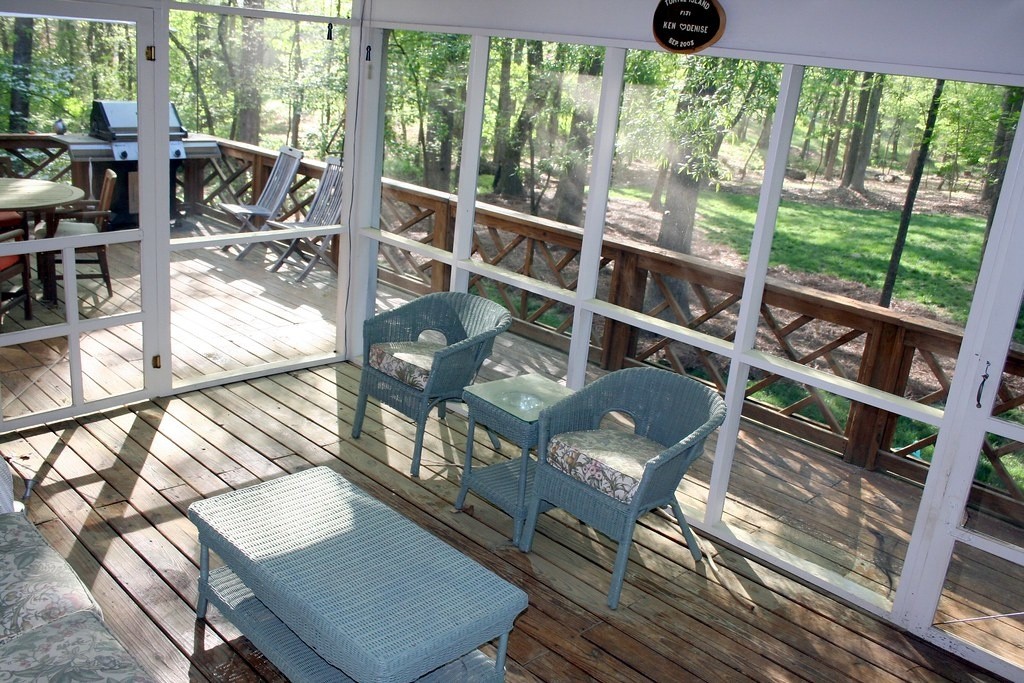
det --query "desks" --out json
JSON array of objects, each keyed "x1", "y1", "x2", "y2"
[
  {"x1": 455, "y1": 372, "x2": 586, "y2": 546},
  {"x1": 0, "y1": 177, "x2": 85, "y2": 300}
]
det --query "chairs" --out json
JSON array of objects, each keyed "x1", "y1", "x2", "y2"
[
  {"x1": 352, "y1": 292, "x2": 511, "y2": 476},
  {"x1": 0, "y1": 229, "x2": 33, "y2": 320},
  {"x1": 266, "y1": 156, "x2": 345, "y2": 280},
  {"x1": 218, "y1": 144, "x2": 304, "y2": 261},
  {"x1": 0, "y1": 157, "x2": 32, "y2": 277},
  {"x1": 39, "y1": 167, "x2": 117, "y2": 305},
  {"x1": 516, "y1": 367, "x2": 727, "y2": 610}
]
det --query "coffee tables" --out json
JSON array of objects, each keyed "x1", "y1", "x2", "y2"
[{"x1": 189, "y1": 465, "x2": 529, "y2": 683}]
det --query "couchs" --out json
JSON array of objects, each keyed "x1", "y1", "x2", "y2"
[{"x1": 0, "y1": 455, "x2": 159, "y2": 683}]
